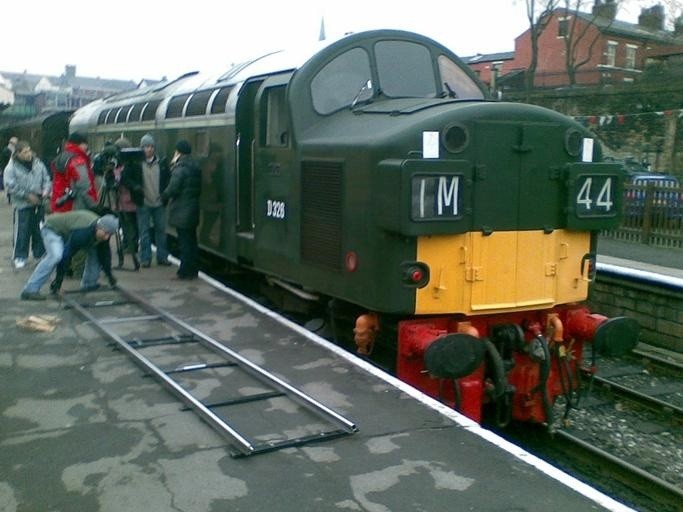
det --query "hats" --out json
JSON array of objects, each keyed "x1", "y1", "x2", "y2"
[
  {"x1": 114, "y1": 135, "x2": 155, "y2": 149},
  {"x1": 97, "y1": 214, "x2": 120, "y2": 233},
  {"x1": 69, "y1": 131, "x2": 87, "y2": 144},
  {"x1": 175, "y1": 139, "x2": 191, "y2": 154}
]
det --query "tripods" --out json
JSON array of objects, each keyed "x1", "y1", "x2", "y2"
[{"x1": 99, "y1": 168, "x2": 141, "y2": 272}]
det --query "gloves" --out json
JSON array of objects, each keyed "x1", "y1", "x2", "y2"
[
  {"x1": 109, "y1": 277, "x2": 117, "y2": 287},
  {"x1": 50, "y1": 276, "x2": 62, "y2": 293}
]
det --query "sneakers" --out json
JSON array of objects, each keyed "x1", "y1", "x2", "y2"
[
  {"x1": 14, "y1": 257, "x2": 26, "y2": 268},
  {"x1": 141, "y1": 258, "x2": 171, "y2": 267}
]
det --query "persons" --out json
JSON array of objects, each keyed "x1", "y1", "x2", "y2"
[
  {"x1": 21, "y1": 209, "x2": 119, "y2": 300},
  {"x1": 0, "y1": 131, "x2": 201, "y2": 279}
]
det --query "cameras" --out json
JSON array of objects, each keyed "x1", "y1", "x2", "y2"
[{"x1": 55, "y1": 187, "x2": 76, "y2": 207}]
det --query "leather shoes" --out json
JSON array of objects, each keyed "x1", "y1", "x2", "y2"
[
  {"x1": 21, "y1": 292, "x2": 47, "y2": 301},
  {"x1": 81, "y1": 284, "x2": 100, "y2": 292}
]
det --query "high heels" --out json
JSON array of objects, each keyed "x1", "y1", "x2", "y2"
[
  {"x1": 177, "y1": 270, "x2": 198, "y2": 280},
  {"x1": 115, "y1": 263, "x2": 140, "y2": 272}
]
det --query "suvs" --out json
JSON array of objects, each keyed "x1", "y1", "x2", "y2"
[{"x1": 618, "y1": 176, "x2": 682, "y2": 226}]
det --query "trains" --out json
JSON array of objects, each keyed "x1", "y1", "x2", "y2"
[{"x1": 0, "y1": 25, "x2": 645, "y2": 436}]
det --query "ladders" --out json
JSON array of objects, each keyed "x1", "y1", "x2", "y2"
[{"x1": 57, "y1": 282, "x2": 360, "y2": 458}]
